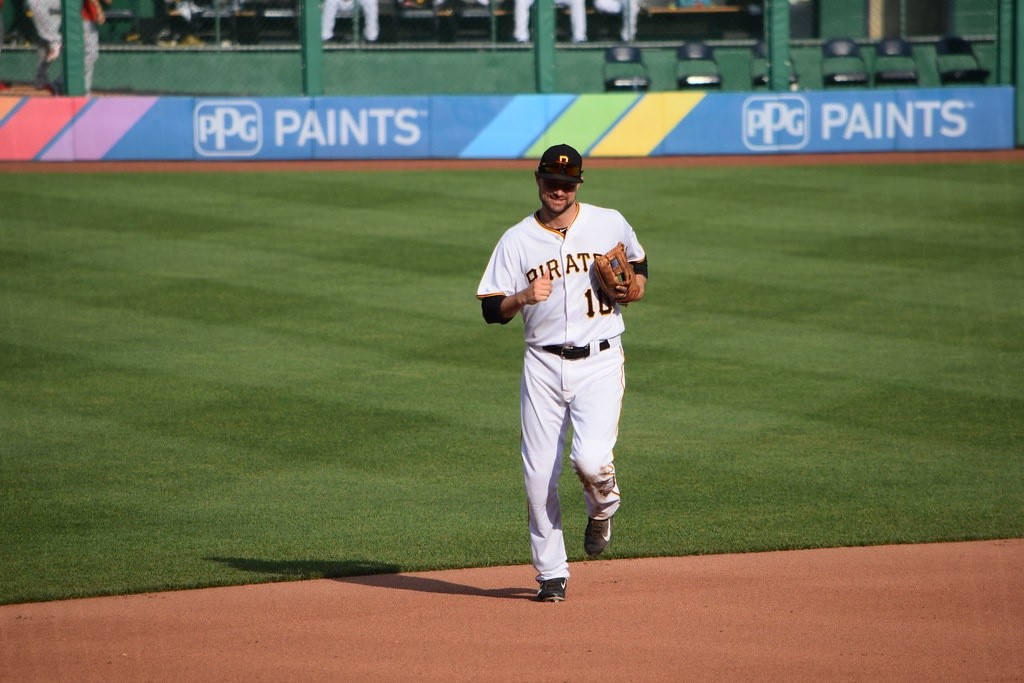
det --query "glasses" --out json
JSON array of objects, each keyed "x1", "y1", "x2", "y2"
[{"x1": 540, "y1": 162, "x2": 582, "y2": 177}]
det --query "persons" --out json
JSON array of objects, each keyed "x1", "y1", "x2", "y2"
[
  {"x1": 476, "y1": 144, "x2": 648, "y2": 603},
  {"x1": 0, "y1": 0, "x2": 111, "y2": 97},
  {"x1": 121, "y1": 0, "x2": 380, "y2": 47},
  {"x1": 514, "y1": 0, "x2": 648, "y2": 43}
]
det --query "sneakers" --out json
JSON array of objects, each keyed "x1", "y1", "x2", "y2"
[
  {"x1": 538, "y1": 577, "x2": 566, "y2": 601},
  {"x1": 584, "y1": 517, "x2": 612, "y2": 555}
]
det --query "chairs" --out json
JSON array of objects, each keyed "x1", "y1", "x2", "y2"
[
  {"x1": 874, "y1": 37, "x2": 919, "y2": 88},
  {"x1": 673, "y1": 41, "x2": 723, "y2": 91},
  {"x1": 99, "y1": 1, "x2": 764, "y2": 41},
  {"x1": 745, "y1": 42, "x2": 801, "y2": 92},
  {"x1": 603, "y1": 45, "x2": 649, "y2": 92},
  {"x1": 821, "y1": 39, "x2": 870, "y2": 88},
  {"x1": 933, "y1": 36, "x2": 987, "y2": 84}
]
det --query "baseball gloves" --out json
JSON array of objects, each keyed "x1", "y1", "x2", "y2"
[{"x1": 593, "y1": 243, "x2": 640, "y2": 305}]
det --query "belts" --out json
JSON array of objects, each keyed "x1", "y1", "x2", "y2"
[{"x1": 543, "y1": 340, "x2": 610, "y2": 360}]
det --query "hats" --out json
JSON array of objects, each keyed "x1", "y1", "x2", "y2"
[{"x1": 538, "y1": 143, "x2": 584, "y2": 183}]
